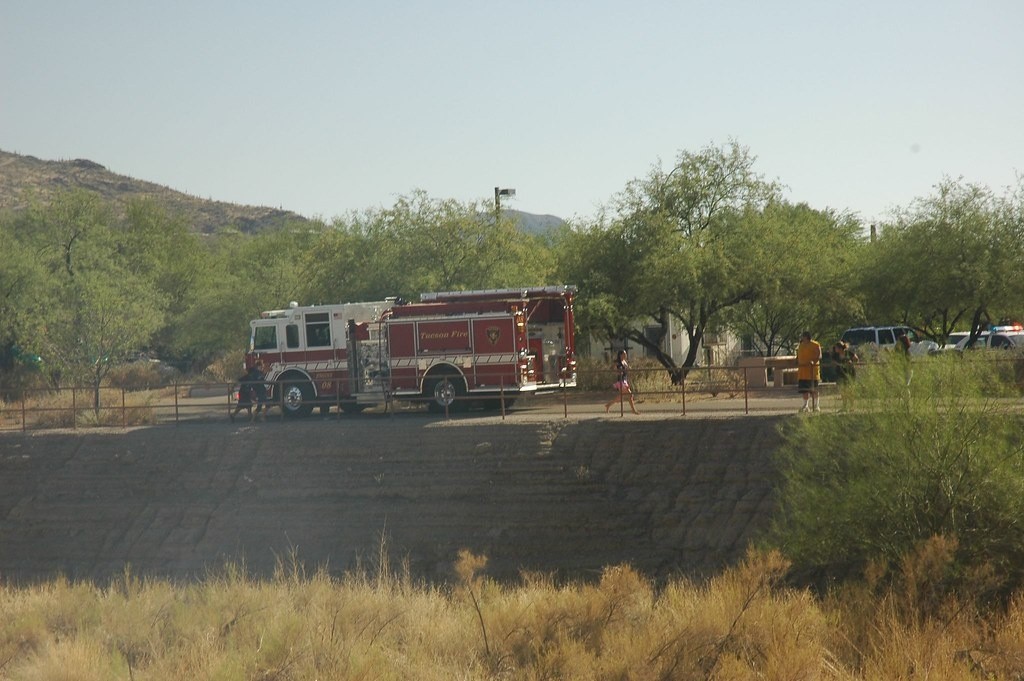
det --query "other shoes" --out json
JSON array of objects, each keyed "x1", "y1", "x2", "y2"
[
  {"x1": 814, "y1": 406, "x2": 820, "y2": 412},
  {"x1": 799, "y1": 407, "x2": 810, "y2": 412},
  {"x1": 230, "y1": 414, "x2": 235, "y2": 422}
]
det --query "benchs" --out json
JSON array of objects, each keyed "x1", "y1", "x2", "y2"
[{"x1": 773, "y1": 367, "x2": 798, "y2": 387}]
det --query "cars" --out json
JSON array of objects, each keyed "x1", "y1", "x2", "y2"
[{"x1": 945, "y1": 325, "x2": 1024, "y2": 351}]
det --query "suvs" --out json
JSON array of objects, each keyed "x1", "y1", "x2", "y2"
[{"x1": 840, "y1": 325, "x2": 939, "y2": 354}]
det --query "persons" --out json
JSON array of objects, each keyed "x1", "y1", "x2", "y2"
[
  {"x1": 832, "y1": 340, "x2": 859, "y2": 414},
  {"x1": 606, "y1": 350, "x2": 641, "y2": 416},
  {"x1": 253, "y1": 363, "x2": 270, "y2": 422},
  {"x1": 231, "y1": 367, "x2": 255, "y2": 424},
  {"x1": 796, "y1": 333, "x2": 821, "y2": 413},
  {"x1": 893, "y1": 334, "x2": 914, "y2": 402}
]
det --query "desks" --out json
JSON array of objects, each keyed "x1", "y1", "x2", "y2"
[{"x1": 738, "y1": 355, "x2": 798, "y2": 387}]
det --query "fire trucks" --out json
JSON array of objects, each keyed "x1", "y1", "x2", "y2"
[{"x1": 243, "y1": 284, "x2": 578, "y2": 417}]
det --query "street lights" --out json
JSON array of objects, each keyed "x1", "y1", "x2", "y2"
[{"x1": 493, "y1": 186, "x2": 517, "y2": 225}]
